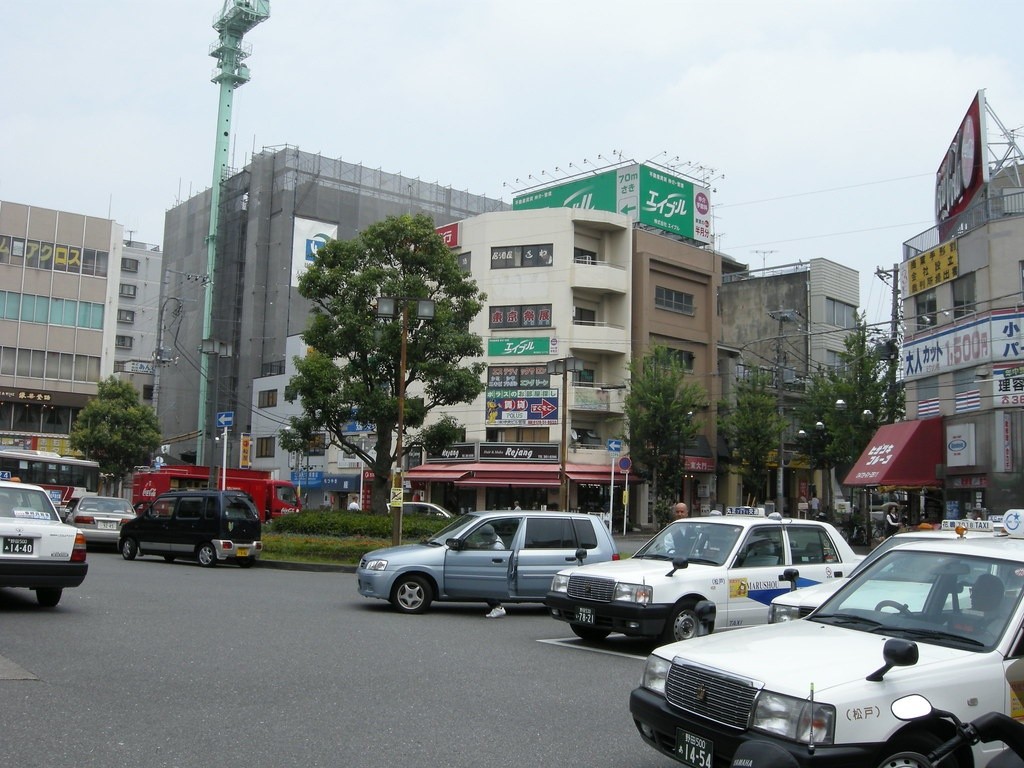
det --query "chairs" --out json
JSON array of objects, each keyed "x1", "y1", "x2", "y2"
[{"x1": 741, "y1": 541, "x2": 781, "y2": 567}]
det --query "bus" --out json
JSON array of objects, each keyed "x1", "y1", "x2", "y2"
[{"x1": 0, "y1": 446, "x2": 100, "y2": 520}]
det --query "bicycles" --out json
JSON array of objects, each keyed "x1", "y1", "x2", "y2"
[{"x1": 870, "y1": 517, "x2": 884, "y2": 539}]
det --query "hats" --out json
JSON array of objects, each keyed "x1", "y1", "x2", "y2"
[{"x1": 479, "y1": 523, "x2": 497, "y2": 540}]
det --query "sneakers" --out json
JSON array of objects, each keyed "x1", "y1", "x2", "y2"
[{"x1": 486, "y1": 606, "x2": 506, "y2": 617}]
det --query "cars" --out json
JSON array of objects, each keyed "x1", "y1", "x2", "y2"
[
  {"x1": 116, "y1": 488, "x2": 265, "y2": 568},
  {"x1": 834, "y1": 525, "x2": 879, "y2": 545},
  {"x1": 0, "y1": 481, "x2": 90, "y2": 608},
  {"x1": 385, "y1": 502, "x2": 454, "y2": 520},
  {"x1": 64, "y1": 496, "x2": 138, "y2": 545},
  {"x1": 767, "y1": 523, "x2": 1024, "y2": 625},
  {"x1": 628, "y1": 535, "x2": 1023, "y2": 768},
  {"x1": 541, "y1": 513, "x2": 894, "y2": 648},
  {"x1": 356, "y1": 508, "x2": 619, "y2": 615}
]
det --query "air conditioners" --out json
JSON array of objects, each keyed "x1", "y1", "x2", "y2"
[{"x1": 576, "y1": 255, "x2": 592, "y2": 264}]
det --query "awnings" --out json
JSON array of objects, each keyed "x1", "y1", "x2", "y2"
[
  {"x1": 401, "y1": 462, "x2": 647, "y2": 489},
  {"x1": 841, "y1": 416, "x2": 945, "y2": 490}
]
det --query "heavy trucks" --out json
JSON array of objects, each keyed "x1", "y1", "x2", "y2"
[{"x1": 131, "y1": 464, "x2": 301, "y2": 523}]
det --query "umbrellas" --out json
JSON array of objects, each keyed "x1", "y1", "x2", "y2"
[{"x1": 880, "y1": 501, "x2": 900, "y2": 508}]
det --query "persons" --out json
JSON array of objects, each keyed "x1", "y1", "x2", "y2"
[
  {"x1": 966, "y1": 509, "x2": 974, "y2": 519},
  {"x1": 475, "y1": 523, "x2": 516, "y2": 618},
  {"x1": 964, "y1": 573, "x2": 1013, "y2": 640},
  {"x1": 885, "y1": 504, "x2": 909, "y2": 540},
  {"x1": 513, "y1": 501, "x2": 522, "y2": 512},
  {"x1": 808, "y1": 492, "x2": 823, "y2": 520},
  {"x1": 663, "y1": 502, "x2": 710, "y2": 560},
  {"x1": 281, "y1": 493, "x2": 291, "y2": 505},
  {"x1": 348, "y1": 495, "x2": 360, "y2": 512},
  {"x1": 411, "y1": 491, "x2": 421, "y2": 503}
]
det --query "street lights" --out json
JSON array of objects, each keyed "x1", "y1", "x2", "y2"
[
  {"x1": 375, "y1": 296, "x2": 436, "y2": 546},
  {"x1": 546, "y1": 356, "x2": 584, "y2": 510}
]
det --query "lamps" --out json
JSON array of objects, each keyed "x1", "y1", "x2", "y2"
[
  {"x1": 598, "y1": 154, "x2": 613, "y2": 165},
  {"x1": 709, "y1": 173, "x2": 726, "y2": 183},
  {"x1": 938, "y1": 308, "x2": 978, "y2": 320},
  {"x1": 502, "y1": 181, "x2": 517, "y2": 193},
  {"x1": 583, "y1": 158, "x2": 598, "y2": 168},
  {"x1": 514, "y1": 178, "x2": 531, "y2": 188},
  {"x1": 698, "y1": 169, "x2": 716, "y2": 179},
  {"x1": 541, "y1": 170, "x2": 556, "y2": 181},
  {"x1": 554, "y1": 166, "x2": 570, "y2": 176},
  {"x1": 663, "y1": 155, "x2": 680, "y2": 165},
  {"x1": 675, "y1": 160, "x2": 692, "y2": 169},
  {"x1": 643, "y1": 150, "x2": 668, "y2": 165},
  {"x1": 688, "y1": 165, "x2": 704, "y2": 174},
  {"x1": 919, "y1": 314, "x2": 958, "y2": 327},
  {"x1": 710, "y1": 187, "x2": 717, "y2": 193},
  {"x1": 899, "y1": 316, "x2": 935, "y2": 335},
  {"x1": 613, "y1": 149, "x2": 628, "y2": 161},
  {"x1": 568, "y1": 162, "x2": 584, "y2": 173},
  {"x1": 528, "y1": 174, "x2": 544, "y2": 185},
  {"x1": 881, "y1": 323, "x2": 915, "y2": 340}
]
